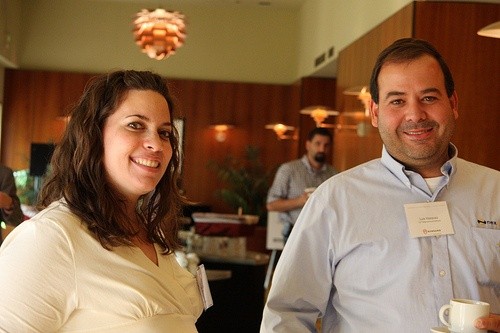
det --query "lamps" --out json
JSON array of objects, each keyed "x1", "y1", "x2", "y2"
[{"x1": 134, "y1": 8, "x2": 187, "y2": 61}]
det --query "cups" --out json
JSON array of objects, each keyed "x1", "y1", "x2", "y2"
[{"x1": 439, "y1": 297, "x2": 490, "y2": 333}]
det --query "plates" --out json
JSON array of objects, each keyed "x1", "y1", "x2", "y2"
[{"x1": 430, "y1": 327, "x2": 450, "y2": 333}]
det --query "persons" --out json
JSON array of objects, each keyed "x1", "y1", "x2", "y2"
[
  {"x1": 0, "y1": 70, "x2": 213, "y2": 333},
  {"x1": 263, "y1": 128, "x2": 341, "y2": 305},
  {"x1": 259, "y1": 38, "x2": 500, "y2": 333},
  {"x1": 0, "y1": 162, "x2": 25, "y2": 224}
]
